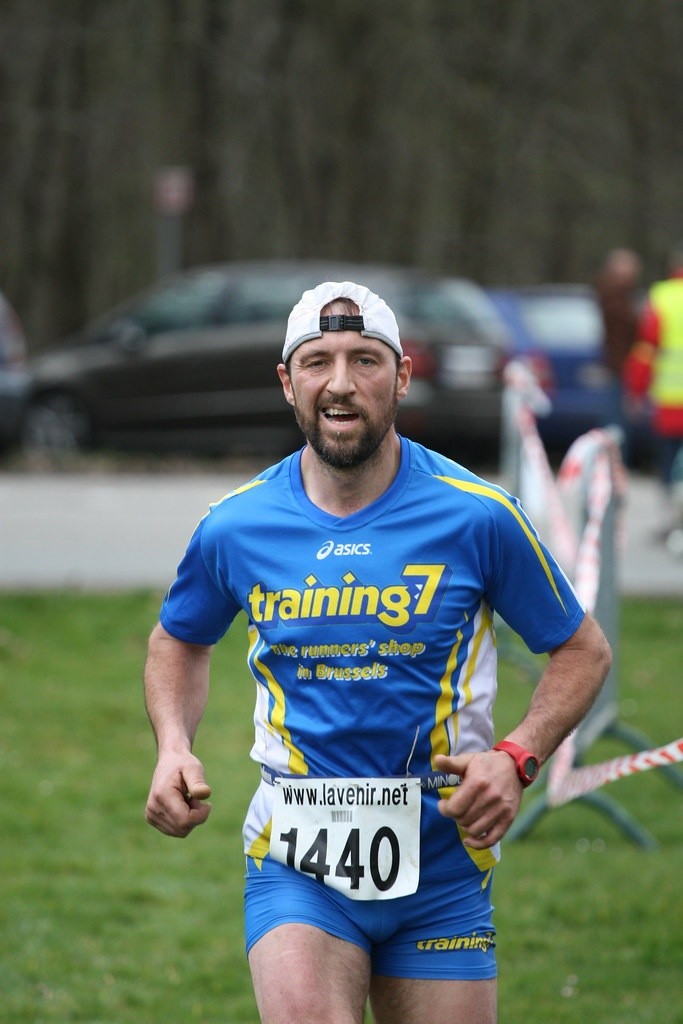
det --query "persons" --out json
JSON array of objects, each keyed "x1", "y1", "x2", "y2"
[
  {"x1": 141, "y1": 280, "x2": 615, "y2": 1024},
  {"x1": 598, "y1": 247, "x2": 683, "y2": 481}
]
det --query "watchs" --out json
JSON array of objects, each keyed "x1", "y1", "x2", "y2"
[{"x1": 494, "y1": 741, "x2": 540, "y2": 788}]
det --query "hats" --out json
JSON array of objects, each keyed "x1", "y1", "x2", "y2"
[{"x1": 281, "y1": 282, "x2": 404, "y2": 362}]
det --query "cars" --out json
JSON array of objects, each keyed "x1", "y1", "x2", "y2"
[{"x1": 492, "y1": 282, "x2": 635, "y2": 433}]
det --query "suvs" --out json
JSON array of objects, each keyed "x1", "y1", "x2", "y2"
[{"x1": 0, "y1": 258, "x2": 516, "y2": 465}]
